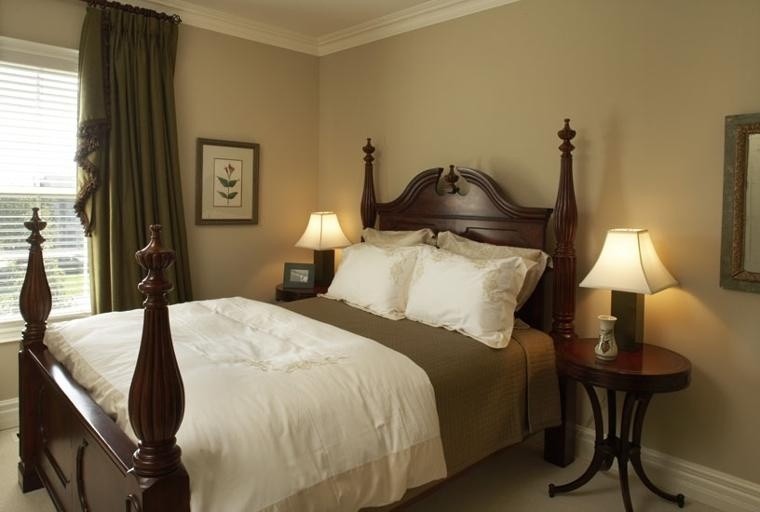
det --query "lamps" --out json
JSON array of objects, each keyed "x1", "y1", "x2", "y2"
[
  {"x1": 294, "y1": 210, "x2": 353, "y2": 288},
  {"x1": 578, "y1": 228, "x2": 680, "y2": 351}
]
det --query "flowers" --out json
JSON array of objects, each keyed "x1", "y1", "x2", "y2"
[{"x1": 216, "y1": 164, "x2": 239, "y2": 206}]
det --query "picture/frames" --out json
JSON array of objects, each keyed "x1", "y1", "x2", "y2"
[
  {"x1": 719, "y1": 112, "x2": 759, "y2": 294},
  {"x1": 195, "y1": 137, "x2": 260, "y2": 225},
  {"x1": 283, "y1": 261, "x2": 316, "y2": 288}
]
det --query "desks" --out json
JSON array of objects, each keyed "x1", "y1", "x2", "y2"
[
  {"x1": 276, "y1": 282, "x2": 331, "y2": 302},
  {"x1": 548, "y1": 338, "x2": 691, "y2": 512}
]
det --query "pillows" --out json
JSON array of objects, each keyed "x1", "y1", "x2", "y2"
[
  {"x1": 436, "y1": 231, "x2": 553, "y2": 330},
  {"x1": 406, "y1": 243, "x2": 538, "y2": 350},
  {"x1": 358, "y1": 227, "x2": 437, "y2": 248},
  {"x1": 316, "y1": 242, "x2": 425, "y2": 322}
]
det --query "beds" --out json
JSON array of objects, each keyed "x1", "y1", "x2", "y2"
[{"x1": 17, "y1": 118, "x2": 578, "y2": 512}]
